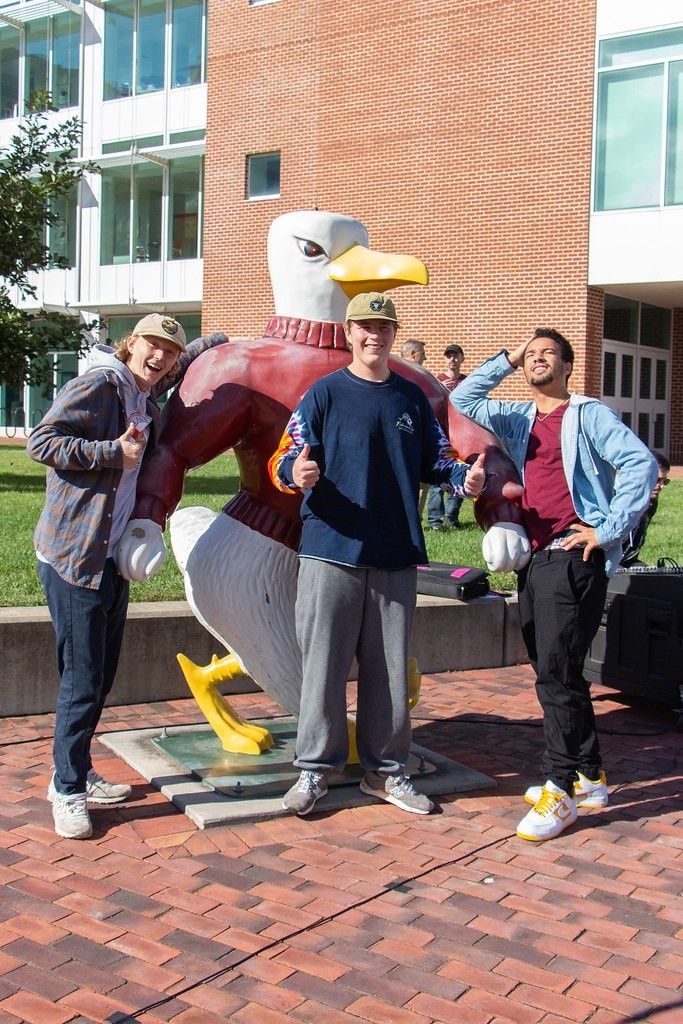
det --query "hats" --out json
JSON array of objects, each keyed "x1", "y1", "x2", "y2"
[
  {"x1": 132, "y1": 313, "x2": 187, "y2": 353},
  {"x1": 443, "y1": 344, "x2": 464, "y2": 356},
  {"x1": 346, "y1": 292, "x2": 400, "y2": 322}
]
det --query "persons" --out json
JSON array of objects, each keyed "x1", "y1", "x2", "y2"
[
  {"x1": 616, "y1": 448, "x2": 670, "y2": 570},
  {"x1": 401, "y1": 339, "x2": 431, "y2": 522},
  {"x1": 424, "y1": 344, "x2": 468, "y2": 534},
  {"x1": 449, "y1": 328, "x2": 659, "y2": 841},
  {"x1": 28, "y1": 311, "x2": 259, "y2": 837},
  {"x1": 268, "y1": 291, "x2": 500, "y2": 816}
]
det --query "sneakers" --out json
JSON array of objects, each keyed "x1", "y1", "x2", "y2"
[
  {"x1": 360, "y1": 770, "x2": 435, "y2": 815},
  {"x1": 524, "y1": 769, "x2": 608, "y2": 808},
  {"x1": 430, "y1": 522, "x2": 443, "y2": 532},
  {"x1": 283, "y1": 769, "x2": 329, "y2": 816},
  {"x1": 46, "y1": 766, "x2": 132, "y2": 804},
  {"x1": 445, "y1": 516, "x2": 461, "y2": 530},
  {"x1": 517, "y1": 779, "x2": 577, "y2": 841},
  {"x1": 51, "y1": 792, "x2": 92, "y2": 839}
]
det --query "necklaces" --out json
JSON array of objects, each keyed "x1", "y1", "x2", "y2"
[{"x1": 536, "y1": 395, "x2": 569, "y2": 422}]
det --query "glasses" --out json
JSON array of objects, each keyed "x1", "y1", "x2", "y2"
[{"x1": 657, "y1": 477, "x2": 670, "y2": 485}]
